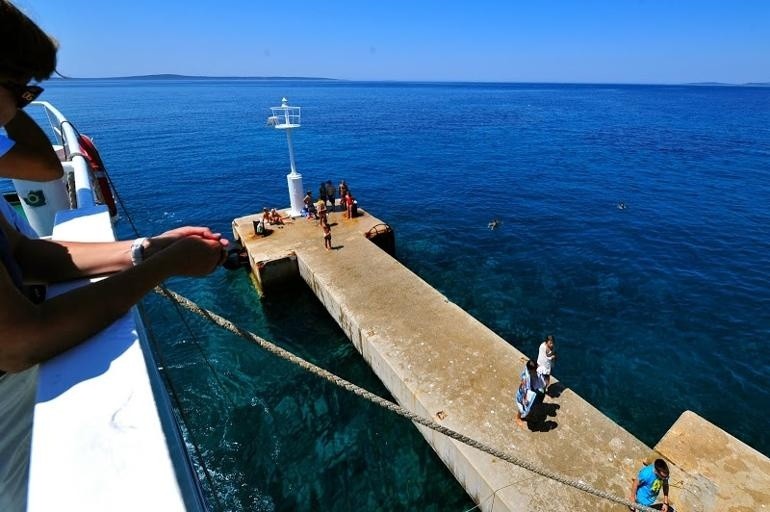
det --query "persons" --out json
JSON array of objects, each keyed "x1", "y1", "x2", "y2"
[
  {"x1": 0, "y1": 0, "x2": 230, "y2": 512},
  {"x1": 488, "y1": 218, "x2": 499, "y2": 230},
  {"x1": 0, "y1": 107, "x2": 64, "y2": 239},
  {"x1": 619, "y1": 203, "x2": 625, "y2": 210},
  {"x1": 302, "y1": 178, "x2": 353, "y2": 228},
  {"x1": 629, "y1": 459, "x2": 670, "y2": 512},
  {"x1": 261, "y1": 206, "x2": 294, "y2": 225},
  {"x1": 535, "y1": 334, "x2": 557, "y2": 386},
  {"x1": 514, "y1": 360, "x2": 546, "y2": 429},
  {"x1": 322, "y1": 218, "x2": 332, "y2": 250}
]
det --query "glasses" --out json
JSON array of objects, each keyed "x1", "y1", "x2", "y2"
[
  {"x1": 658, "y1": 472, "x2": 669, "y2": 480},
  {"x1": 16, "y1": 86, "x2": 44, "y2": 109}
]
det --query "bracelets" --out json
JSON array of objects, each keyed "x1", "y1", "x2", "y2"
[
  {"x1": 664, "y1": 502, "x2": 669, "y2": 507},
  {"x1": 130, "y1": 234, "x2": 145, "y2": 268}
]
